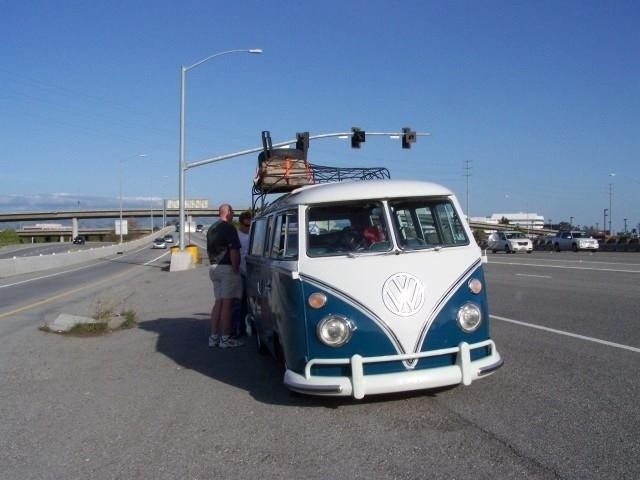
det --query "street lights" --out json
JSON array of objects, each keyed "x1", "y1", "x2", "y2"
[
  {"x1": 118, "y1": 154, "x2": 144, "y2": 242},
  {"x1": 149, "y1": 175, "x2": 168, "y2": 233},
  {"x1": 178, "y1": 47, "x2": 262, "y2": 250}
]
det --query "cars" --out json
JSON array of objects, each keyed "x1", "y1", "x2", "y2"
[
  {"x1": 164, "y1": 235, "x2": 172, "y2": 243},
  {"x1": 153, "y1": 238, "x2": 166, "y2": 249},
  {"x1": 196, "y1": 224, "x2": 203, "y2": 231},
  {"x1": 488, "y1": 230, "x2": 533, "y2": 254},
  {"x1": 551, "y1": 230, "x2": 599, "y2": 251},
  {"x1": 73, "y1": 236, "x2": 86, "y2": 245}
]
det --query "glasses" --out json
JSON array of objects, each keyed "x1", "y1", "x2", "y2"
[{"x1": 243, "y1": 223, "x2": 250, "y2": 227}]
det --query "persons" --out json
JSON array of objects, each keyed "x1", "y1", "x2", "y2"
[
  {"x1": 207, "y1": 204, "x2": 245, "y2": 348},
  {"x1": 229, "y1": 212, "x2": 252, "y2": 339}
]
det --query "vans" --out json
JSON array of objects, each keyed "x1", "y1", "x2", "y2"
[{"x1": 242, "y1": 178, "x2": 505, "y2": 398}]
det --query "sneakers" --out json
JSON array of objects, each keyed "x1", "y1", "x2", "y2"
[
  {"x1": 219, "y1": 335, "x2": 245, "y2": 348},
  {"x1": 209, "y1": 335, "x2": 220, "y2": 348},
  {"x1": 239, "y1": 331, "x2": 248, "y2": 336},
  {"x1": 231, "y1": 330, "x2": 237, "y2": 338}
]
